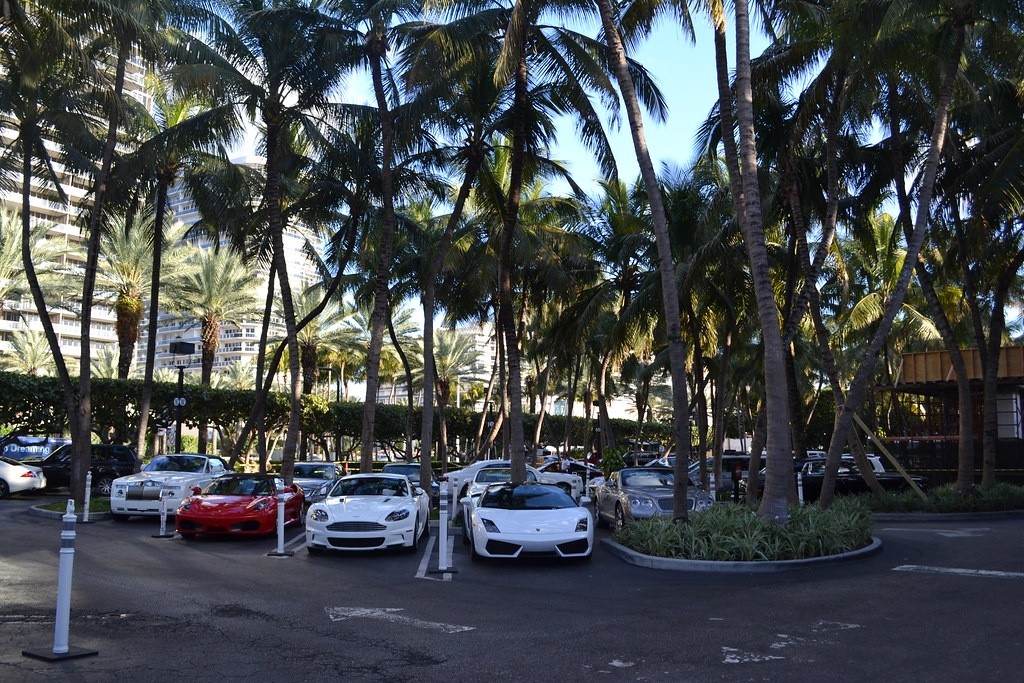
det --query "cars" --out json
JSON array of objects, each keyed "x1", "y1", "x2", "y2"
[{"x1": 0, "y1": 435, "x2": 929, "y2": 565}]
